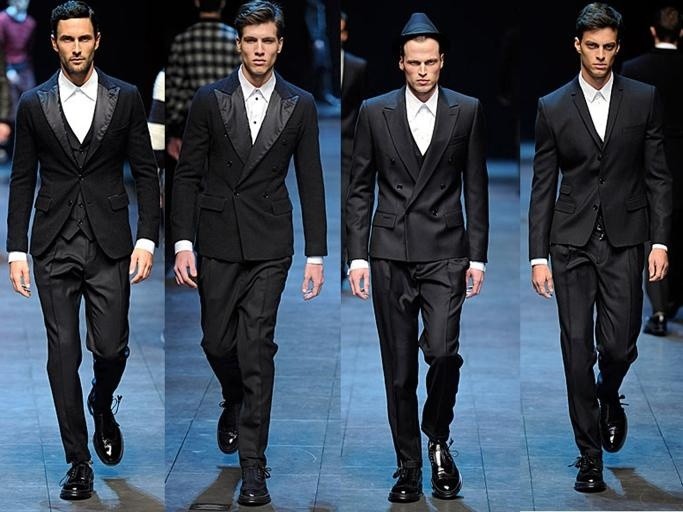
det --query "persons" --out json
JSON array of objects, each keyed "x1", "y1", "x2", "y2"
[
  {"x1": 526, "y1": 1, "x2": 674, "y2": 489},
  {"x1": 340, "y1": 15, "x2": 372, "y2": 284},
  {"x1": 344, "y1": 34, "x2": 492, "y2": 503},
  {"x1": 164, "y1": 1, "x2": 243, "y2": 233},
  {"x1": 619, "y1": 3, "x2": 682, "y2": 338},
  {"x1": 3, "y1": 0, "x2": 161, "y2": 502},
  {"x1": 169, "y1": 0, "x2": 329, "y2": 508},
  {"x1": 0, "y1": 0, "x2": 37, "y2": 157}
]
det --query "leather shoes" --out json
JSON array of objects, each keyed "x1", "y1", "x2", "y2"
[
  {"x1": 87, "y1": 393, "x2": 124, "y2": 465},
  {"x1": 387, "y1": 466, "x2": 424, "y2": 504},
  {"x1": 216, "y1": 399, "x2": 239, "y2": 454},
  {"x1": 599, "y1": 390, "x2": 628, "y2": 452},
  {"x1": 236, "y1": 466, "x2": 270, "y2": 507},
  {"x1": 427, "y1": 438, "x2": 464, "y2": 499},
  {"x1": 574, "y1": 454, "x2": 608, "y2": 495},
  {"x1": 59, "y1": 462, "x2": 95, "y2": 501},
  {"x1": 643, "y1": 310, "x2": 669, "y2": 335}
]
